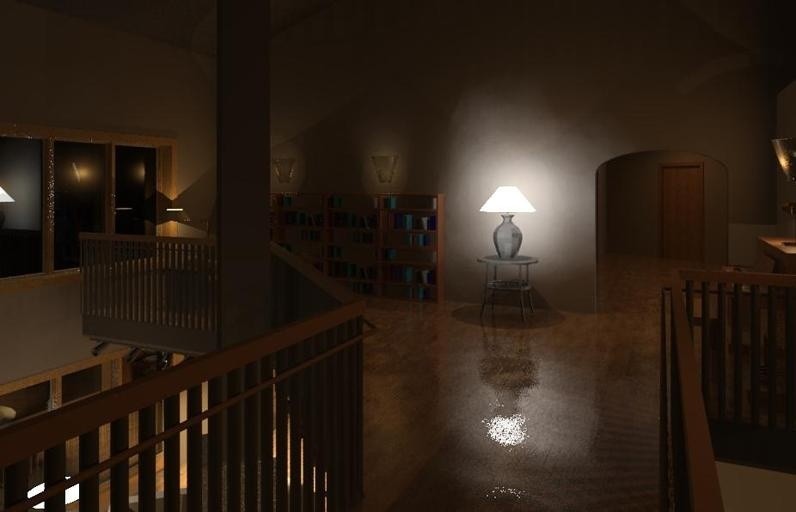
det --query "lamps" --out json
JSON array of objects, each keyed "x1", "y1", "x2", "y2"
[
  {"x1": 0, "y1": 186, "x2": 15, "y2": 231},
  {"x1": 272, "y1": 158, "x2": 296, "y2": 183},
  {"x1": 157, "y1": 207, "x2": 192, "y2": 248},
  {"x1": 769, "y1": 136, "x2": 796, "y2": 182},
  {"x1": 371, "y1": 153, "x2": 401, "y2": 183},
  {"x1": 479, "y1": 185, "x2": 537, "y2": 259}
]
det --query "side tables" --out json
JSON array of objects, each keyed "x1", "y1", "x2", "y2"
[{"x1": 475, "y1": 255, "x2": 539, "y2": 323}]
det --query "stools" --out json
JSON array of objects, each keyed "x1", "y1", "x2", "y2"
[{"x1": 720, "y1": 265, "x2": 757, "y2": 330}]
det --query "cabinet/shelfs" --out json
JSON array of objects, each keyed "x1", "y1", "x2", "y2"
[{"x1": 270, "y1": 191, "x2": 446, "y2": 307}]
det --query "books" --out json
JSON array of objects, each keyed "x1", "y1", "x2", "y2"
[
  {"x1": 278, "y1": 192, "x2": 323, "y2": 257},
  {"x1": 382, "y1": 195, "x2": 437, "y2": 302},
  {"x1": 328, "y1": 193, "x2": 379, "y2": 296}
]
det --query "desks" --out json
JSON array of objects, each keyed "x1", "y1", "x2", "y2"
[{"x1": 757, "y1": 234, "x2": 796, "y2": 298}]
